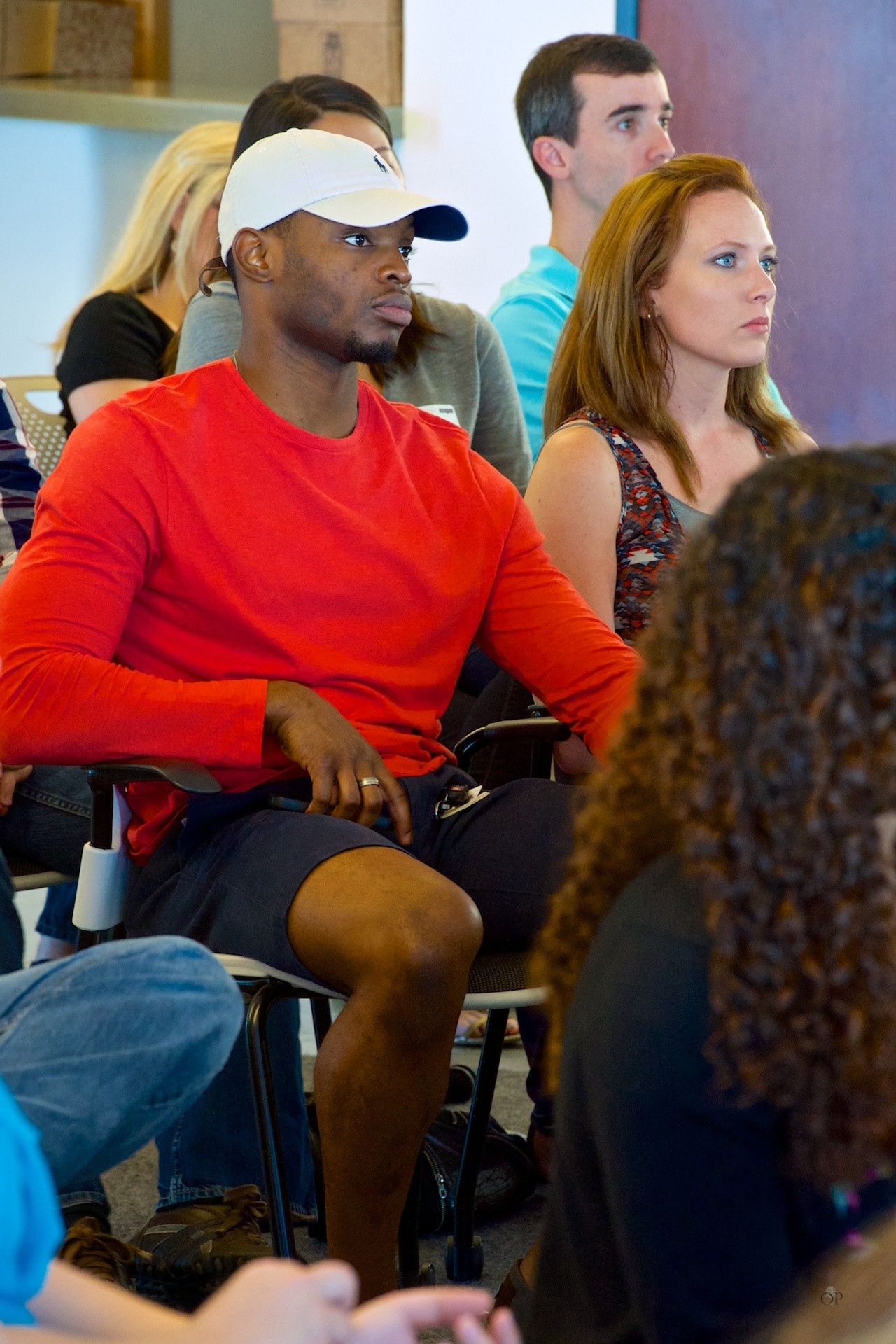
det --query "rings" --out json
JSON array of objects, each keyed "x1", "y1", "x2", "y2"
[{"x1": 358, "y1": 776, "x2": 381, "y2": 788}]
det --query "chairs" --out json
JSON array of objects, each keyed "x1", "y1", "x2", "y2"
[{"x1": 0, "y1": 376, "x2": 574, "y2": 1278}]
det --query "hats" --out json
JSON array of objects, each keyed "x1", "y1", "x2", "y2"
[{"x1": 216, "y1": 127, "x2": 469, "y2": 267}]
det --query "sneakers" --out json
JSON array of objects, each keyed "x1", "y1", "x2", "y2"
[
  {"x1": 127, "y1": 1176, "x2": 318, "y2": 1301},
  {"x1": 55, "y1": 1216, "x2": 162, "y2": 1304}
]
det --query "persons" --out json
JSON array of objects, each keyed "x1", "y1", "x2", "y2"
[{"x1": 0, "y1": 33, "x2": 895, "y2": 1343}]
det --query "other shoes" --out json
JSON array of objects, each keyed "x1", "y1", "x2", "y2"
[{"x1": 496, "y1": 1259, "x2": 568, "y2": 1344}]
